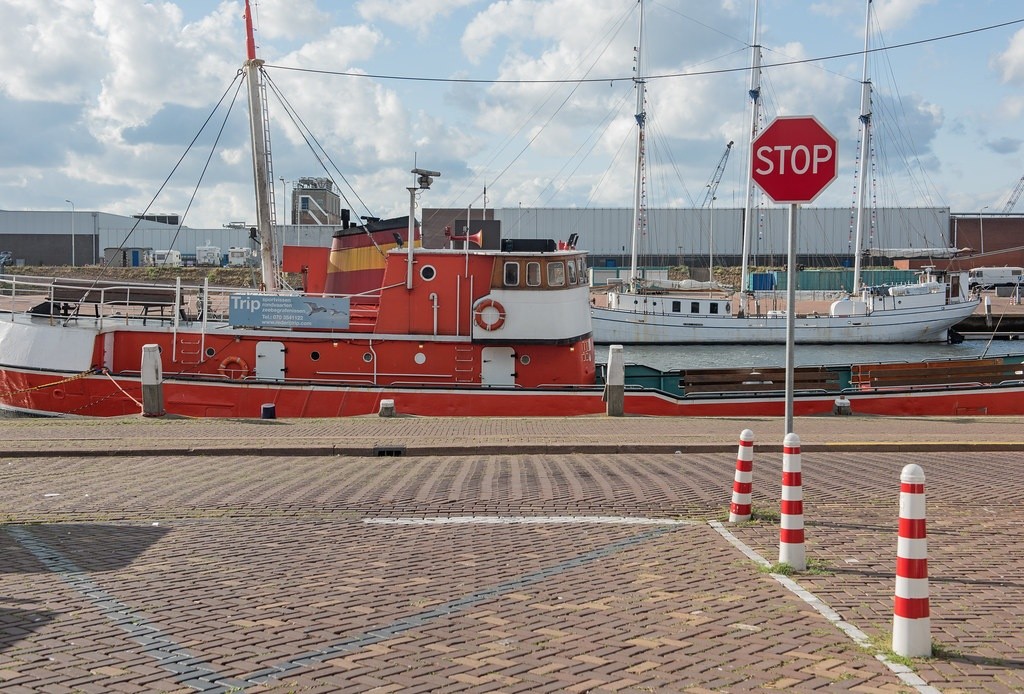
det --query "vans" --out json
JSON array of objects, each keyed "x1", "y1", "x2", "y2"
[{"x1": 969, "y1": 266, "x2": 1024, "y2": 291}]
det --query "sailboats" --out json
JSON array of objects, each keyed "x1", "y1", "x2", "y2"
[{"x1": 589, "y1": 0, "x2": 985, "y2": 347}]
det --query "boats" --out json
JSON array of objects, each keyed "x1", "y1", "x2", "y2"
[{"x1": 0, "y1": 0, "x2": 1024, "y2": 421}]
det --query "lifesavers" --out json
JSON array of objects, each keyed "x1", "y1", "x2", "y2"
[
  {"x1": 474, "y1": 299, "x2": 506, "y2": 332},
  {"x1": 217, "y1": 355, "x2": 249, "y2": 384}
]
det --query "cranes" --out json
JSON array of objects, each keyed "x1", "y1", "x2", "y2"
[
  {"x1": 1001, "y1": 174, "x2": 1024, "y2": 214},
  {"x1": 696, "y1": 140, "x2": 734, "y2": 208}
]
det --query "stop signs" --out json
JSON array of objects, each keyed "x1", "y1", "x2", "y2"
[{"x1": 750, "y1": 115, "x2": 839, "y2": 204}]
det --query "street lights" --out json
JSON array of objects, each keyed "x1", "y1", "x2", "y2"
[
  {"x1": 65, "y1": 199, "x2": 75, "y2": 267},
  {"x1": 980, "y1": 206, "x2": 989, "y2": 255}
]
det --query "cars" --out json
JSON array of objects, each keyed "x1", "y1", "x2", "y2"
[{"x1": 0, "y1": 250, "x2": 14, "y2": 266}]
